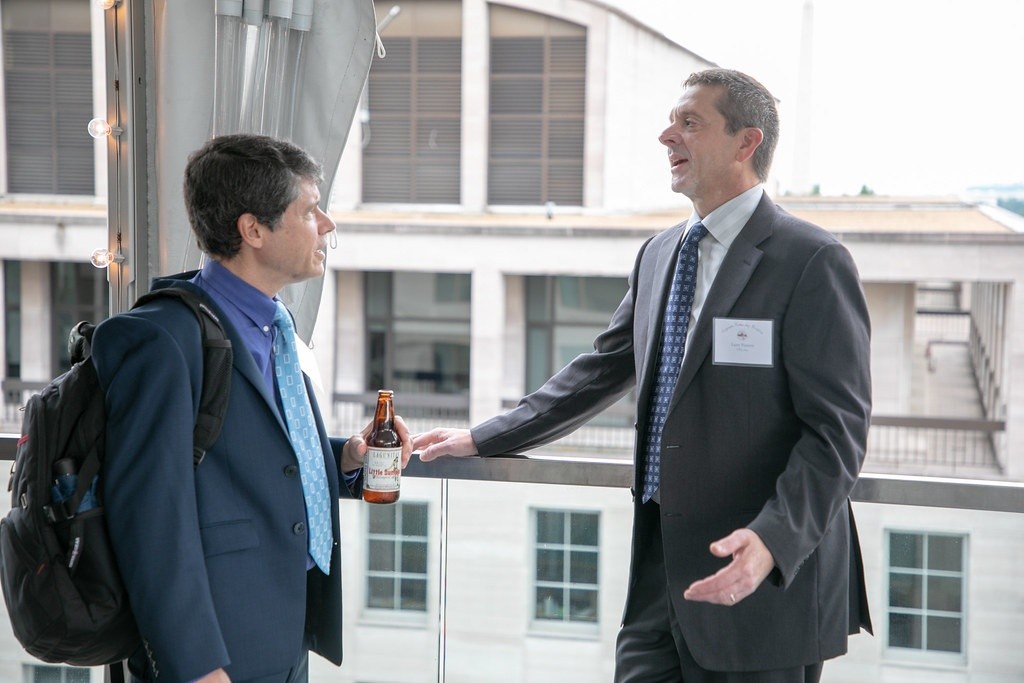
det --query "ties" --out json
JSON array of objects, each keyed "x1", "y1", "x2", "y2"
[
  {"x1": 268, "y1": 301, "x2": 335, "y2": 576},
  {"x1": 640, "y1": 222, "x2": 709, "y2": 504}
]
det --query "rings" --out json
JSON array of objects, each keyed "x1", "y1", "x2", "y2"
[{"x1": 730, "y1": 594, "x2": 735, "y2": 602}]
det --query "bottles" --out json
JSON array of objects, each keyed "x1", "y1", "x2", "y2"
[{"x1": 362, "y1": 390, "x2": 402, "y2": 503}]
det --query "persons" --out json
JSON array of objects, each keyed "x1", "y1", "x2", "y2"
[
  {"x1": 412, "y1": 69, "x2": 874, "y2": 683},
  {"x1": 91, "y1": 135, "x2": 414, "y2": 683}
]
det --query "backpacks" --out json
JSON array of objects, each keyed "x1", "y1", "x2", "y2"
[{"x1": 0, "y1": 286, "x2": 233, "y2": 666}]
